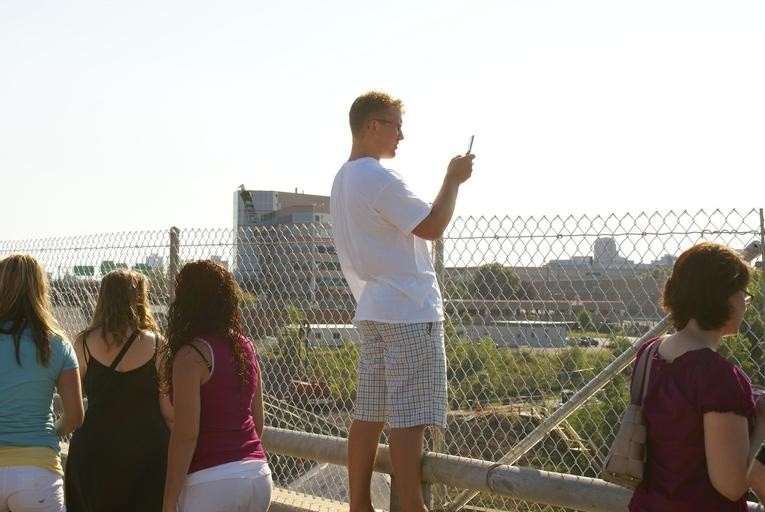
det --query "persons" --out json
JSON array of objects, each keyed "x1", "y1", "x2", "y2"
[
  {"x1": 330, "y1": 91, "x2": 476, "y2": 512},
  {"x1": 627, "y1": 241, "x2": 765, "y2": 512}
]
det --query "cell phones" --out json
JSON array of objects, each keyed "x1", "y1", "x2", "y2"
[{"x1": 466, "y1": 135, "x2": 474, "y2": 155}]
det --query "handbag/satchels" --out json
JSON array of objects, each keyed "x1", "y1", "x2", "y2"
[{"x1": 602, "y1": 334, "x2": 673, "y2": 490}]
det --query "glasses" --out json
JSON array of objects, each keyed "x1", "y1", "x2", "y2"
[{"x1": 373, "y1": 119, "x2": 402, "y2": 131}]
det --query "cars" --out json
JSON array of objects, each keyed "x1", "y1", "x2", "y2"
[{"x1": 580, "y1": 337, "x2": 599, "y2": 347}]
